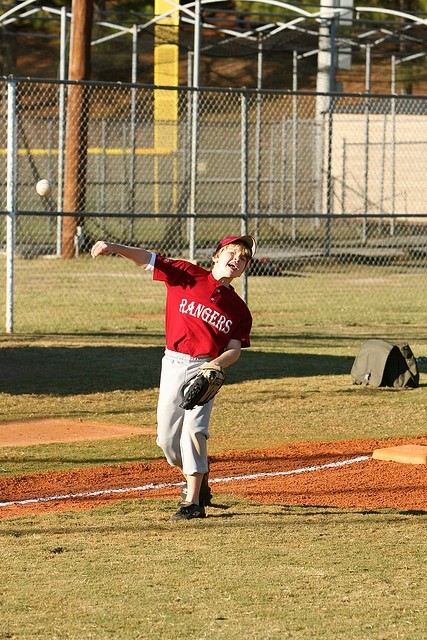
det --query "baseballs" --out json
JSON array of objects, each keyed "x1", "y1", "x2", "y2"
[{"x1": 36, "y1": 179, "x2": 50, "y2": 196}]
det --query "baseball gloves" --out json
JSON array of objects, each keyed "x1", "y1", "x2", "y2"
[{"x1": 179, "y1": 362, "x2": 227, "y2": 410}]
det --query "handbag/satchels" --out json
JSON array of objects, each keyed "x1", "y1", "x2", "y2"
[{"x1": 351, "y1": 339, "x2": 419, "y2": 388}]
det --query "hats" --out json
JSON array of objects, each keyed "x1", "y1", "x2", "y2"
[{"x1": 216, "y1": 235, "x2": 257, "y2": 258}]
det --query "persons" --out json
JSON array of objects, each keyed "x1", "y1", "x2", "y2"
[
  {"x1": 74, "y1": 222, "x2": 93, "y2": 258},
  {"x1": 91, "y1": 234, "x2": 257, "y2": 523}
]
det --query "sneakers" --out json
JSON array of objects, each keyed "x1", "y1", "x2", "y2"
[
  {"x1": 167, "y1": 501, "x2": 206, "y2": 520},
  {"x1": 201, "y1": 460, "x2": 213, "y2": 507}
]
